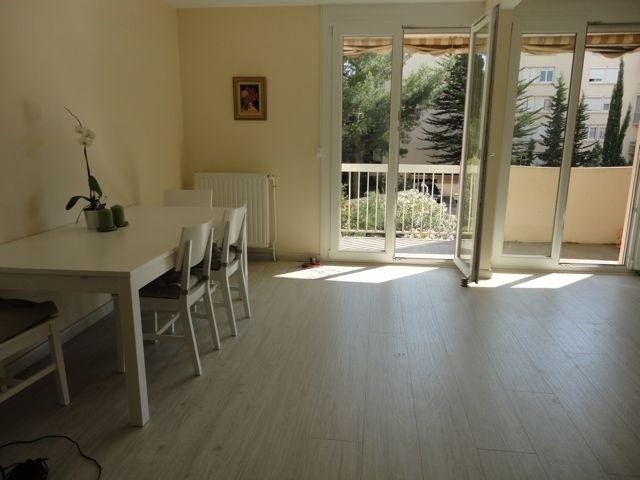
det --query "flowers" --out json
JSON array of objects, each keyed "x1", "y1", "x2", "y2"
[{"x1": 59, "y1": 104, "x2": 107, "y2": 224}]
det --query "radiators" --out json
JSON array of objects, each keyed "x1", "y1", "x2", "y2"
[{"x1": 192, "y1": 173, "x2": 269, "y2": 247}]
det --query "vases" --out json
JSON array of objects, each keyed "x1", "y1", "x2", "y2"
[{"x1": 83, "y1": 209, "x2": 101, "y2": 231}]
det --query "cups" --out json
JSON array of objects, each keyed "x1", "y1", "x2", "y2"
[
  {"x1": 111, "y1": 204, "x2": 125, "y2": 224},
  {"x1": 98, "y1": 208, "x2": 113, "y2": 228}
]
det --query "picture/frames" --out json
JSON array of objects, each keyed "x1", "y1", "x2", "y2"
[{"x1": 231, "y1": 77, "x2": 267, "y2": 121}]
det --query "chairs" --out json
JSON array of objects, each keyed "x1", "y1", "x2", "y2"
[
  {"x1": 113, "y1": 219, "x2": 221, "y2": 377},
  {"x1": 0, "y1": 298, "x2": 70, "y2": 408},
  {"x1": 210, "y1": 202, "x2": 254, "y2": 337},
  {"x1": 164, "y1": 190, "x2": 212, "y2": 207}
]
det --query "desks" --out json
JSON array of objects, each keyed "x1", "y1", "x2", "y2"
[{"x1": 0, "y1": 209, "x2": 248, "y2": 427}]
